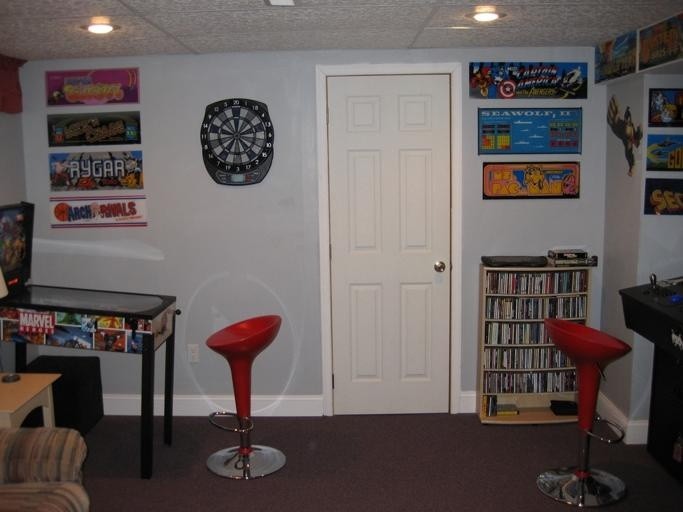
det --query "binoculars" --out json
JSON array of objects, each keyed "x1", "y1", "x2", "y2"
[{"x1": 200, "y1": 98, "x2": 274, "y2": 184}]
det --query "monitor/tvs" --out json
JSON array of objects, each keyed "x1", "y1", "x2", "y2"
[{"x1": 481, "y1": 248, "x2": 598, "y2": 417}]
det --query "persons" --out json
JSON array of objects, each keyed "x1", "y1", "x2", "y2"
[{"x1": 145, "y1": 322, "x2": 152, "y2": 330}]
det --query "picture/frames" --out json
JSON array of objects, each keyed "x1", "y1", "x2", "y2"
[{"x1": 0, "y1": 371, "x2": 67, "y2": 432}]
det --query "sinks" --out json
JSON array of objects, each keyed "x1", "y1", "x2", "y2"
[{"x1": 0, "y1": 425, "x2": 93, "y2": 512}]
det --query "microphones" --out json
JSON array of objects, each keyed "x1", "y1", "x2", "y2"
[
  {"x1": 202, "y1": 312, "x2": 286, "y2": 483},
  {"x1": 532, "y1": 311, "x2": 633, "y2": 512}
]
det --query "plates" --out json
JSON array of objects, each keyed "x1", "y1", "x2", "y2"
[{"x1": 474, "y1": 260, "x2": 593, "y2": 425}]
